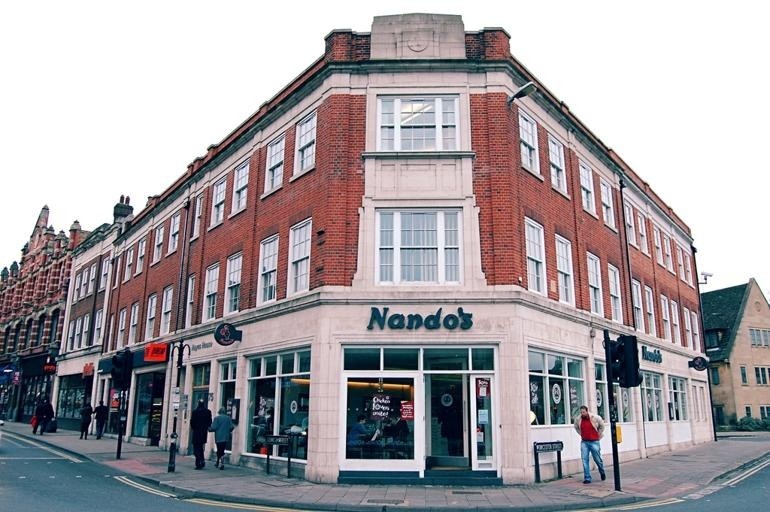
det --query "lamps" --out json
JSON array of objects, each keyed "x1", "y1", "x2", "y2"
[
  {"x1": 46, "y1": 356, "x2": 58, "y2": 366},
  {"x1": 508, "y1": 82, "x2": 538, "y2": 108}
]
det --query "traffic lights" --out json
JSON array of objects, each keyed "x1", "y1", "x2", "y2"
[{"x1": 111, "y1": 350, "x2": 134, "y2": 392}]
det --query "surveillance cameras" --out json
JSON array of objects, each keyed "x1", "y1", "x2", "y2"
[
  {"x1": 701, "y1": 271, "x2": 713, "y2": 277},
  {"x1": 518, "y1": 276, "x2": 522, "y2": 284}
]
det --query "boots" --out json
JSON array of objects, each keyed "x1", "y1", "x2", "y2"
[{"x1": 214, "y1": 456, "x2": 225, "y2": 470}]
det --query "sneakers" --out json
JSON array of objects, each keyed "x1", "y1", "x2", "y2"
[
  {"x1": 600, "y1": 473, "x2": 606, "y2": 480},
  {"x1": 194, "y1": 462, "x2": 205, "y2": 470},
  {"x1": 582, "y1": 480, "x2": 592, "y2": 484}
]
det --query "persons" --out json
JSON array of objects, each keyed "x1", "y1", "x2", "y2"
[
  {"x1": 93, "y1": 400, "x2": 109, "y2": 439},
  {"x1": 189, "y1": 397, "x2": 212, "y2": 470},
  {"x1": 79, "y1": 402, "x2": 93, "y2": 441},
  {"x1": 438, "y1": 386, "x2": 463, "y2": 455},
  {"x1": 208, "y1": 406, "x2": 235, "y2": 470},
  {"x1": 33, "y1": 398, "x2": 54, "y2": 436},
  {"x1": 574, "y1": 403, "x2": 607, "y2": 485},
  {"x1": 348, "y1": 412, "x2": 410, "y2": 459}
]
